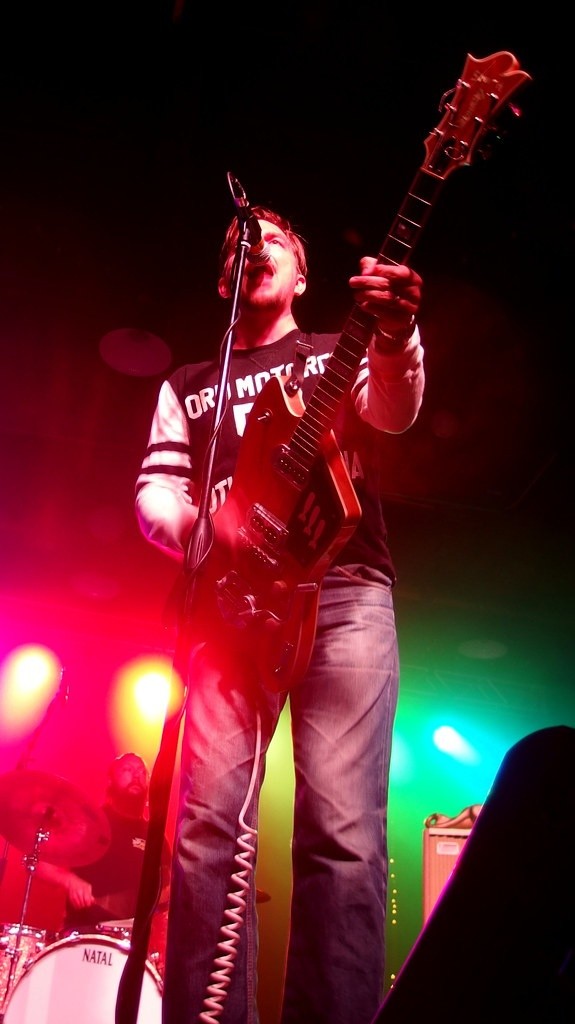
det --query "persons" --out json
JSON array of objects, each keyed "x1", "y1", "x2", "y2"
[
  {"x1": 30, "y1": 751, "x2": 173, "y2": 938},
  {"x1": 130, "y1": 207, "x2": 424, "y2": 1024}
]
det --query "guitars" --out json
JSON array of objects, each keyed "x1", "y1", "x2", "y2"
[{"x1": 166, "y1": 50, "x2": 529, "y2": 694}]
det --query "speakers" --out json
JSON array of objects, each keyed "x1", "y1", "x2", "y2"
[{"x1": 371, "y1": 725, "x2": 575, "y2": 1024}]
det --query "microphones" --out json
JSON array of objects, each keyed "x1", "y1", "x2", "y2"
[{"x1": 229, "y1": 175, "x2": 271, "y2": 266}]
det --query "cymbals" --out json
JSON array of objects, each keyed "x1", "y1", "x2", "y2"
[
  {"x1": 1, "y1": 766, "x2": 111, "y2": 868},
  {"x1": 256, "y1": 886, "x2": 272, "y2": 907}
]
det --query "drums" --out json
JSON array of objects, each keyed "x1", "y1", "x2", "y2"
[
  {"x1": 2, "y1": 931, "x2": 162, "y2": 1024},
  {"x1": 97, "y1": 917, "x2": 134, "y2": 940},
  {"x1": 0, "y1": 919, "x2": 44, "y2": 1014}
]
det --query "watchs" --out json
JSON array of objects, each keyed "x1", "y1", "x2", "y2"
[{"x1": 373, "y1": 312, "x2": 417, "y2": 350}]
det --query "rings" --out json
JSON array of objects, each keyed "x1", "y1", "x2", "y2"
[{"x1": 390, "y1": 294, "x2": 404, "y2": 305}]
